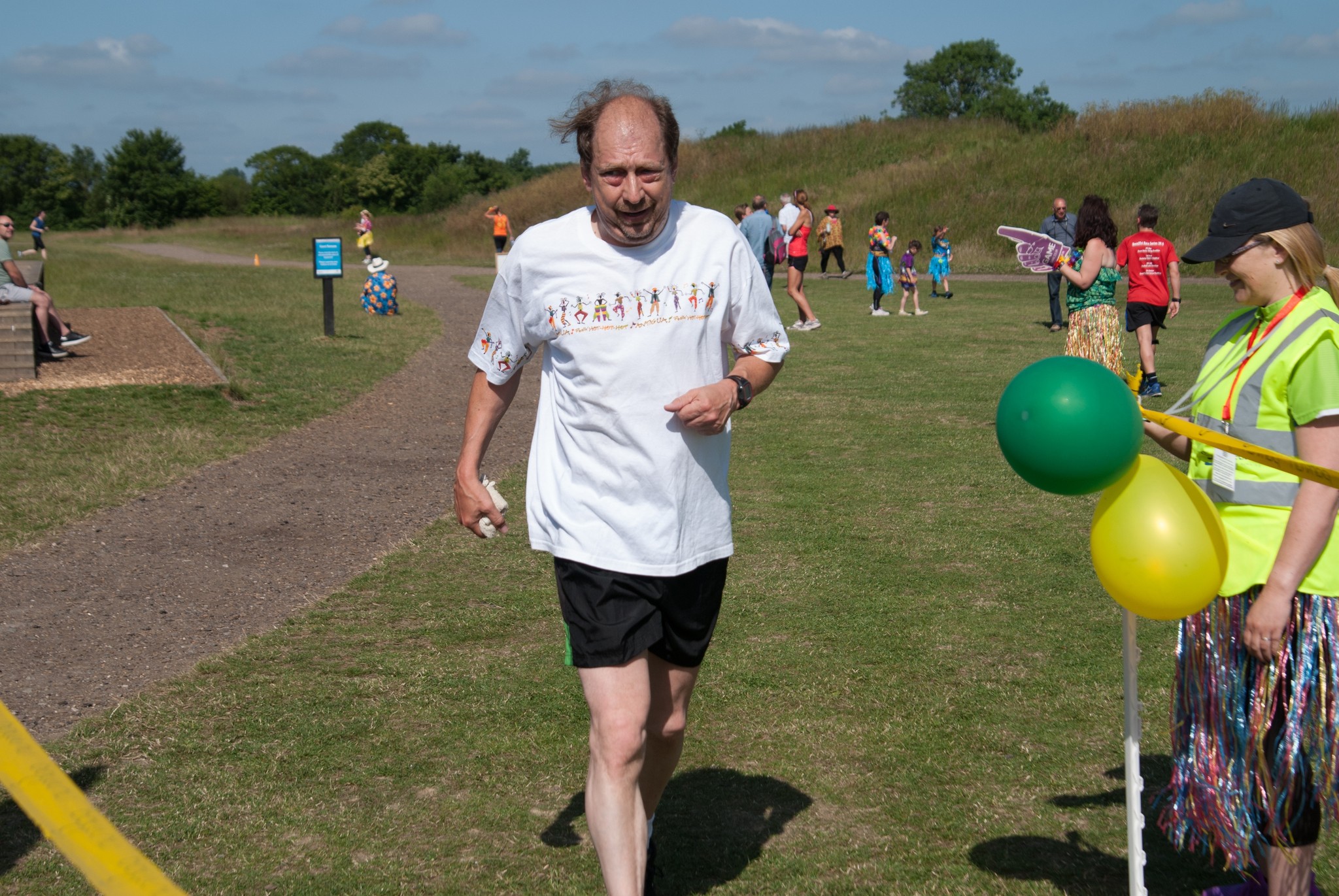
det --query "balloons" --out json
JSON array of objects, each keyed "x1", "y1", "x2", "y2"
[
  {"x1": 1089, "y1": 454, "x2": 1229, "y2": 621},
  {"x1": 994, "y1": 355, "x2": 1144, "y2": 495}
]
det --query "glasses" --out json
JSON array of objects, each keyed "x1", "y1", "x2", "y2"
[
  {"x1": 0, "y1": 223, "x2": 14, "y2": 227},
  {"x1": 1219, "y1": 240, "x2": 1263, "y2": 264},
  {"x1": 1057, "y1": 207, "x2": 1066, "y2": 210}
]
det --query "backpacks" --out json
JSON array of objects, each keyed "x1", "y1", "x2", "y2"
[{"x1": 764, "y1": 216, "x2": 787, "y2": 264}]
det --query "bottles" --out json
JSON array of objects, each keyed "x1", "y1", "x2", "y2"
[{"x1": 826, "y1": 223, "x2": 831, "y2": 233}]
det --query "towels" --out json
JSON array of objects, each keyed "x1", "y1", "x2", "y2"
[{"x1": 478, "y1": 475, "x2": 508, "y2": 539}]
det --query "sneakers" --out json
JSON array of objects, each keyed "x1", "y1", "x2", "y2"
[
  {"x1": 786, "y1": 320, "x2": 807, "y2": 330},
  {"x1": 37, "y1": 341, "x2": 68, "y2": 358},
  {"x1": 1140, "y1": 382, "x2": 1162, "y2": 396},
  {"x1": 872, "y1": 309, "x2": 889, "y2": 316},
  {"x1": 870, "y1": 303, "x2": 883, "y2": 311},
  {"x1": 60, "y1": 331, "x2": 91, "y2": 346},
  {"x1": 1140, "y1": 378, "x2": 1147, "y2": 391},
  {"x1": 798, "y1": 319, "x2": 821, "y2": 331}
]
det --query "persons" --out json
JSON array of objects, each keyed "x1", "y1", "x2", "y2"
[
  {"x1": 733, "y1": 189, "x2": 821, "y2": 332},
  {"x1": 453, "y1": 80, "x2": 791, "y2": 896},
  {"x1": 1134, "y1": 175, "x2": 1339, "y2": 896},
  {"x1": 929, "y1": 225, "x2": 954, "y2": 299},
  {"x1": 360, "y1": 257, "x2": 398, "y2": 316},
  {"x1": 353, "y1": 209, "x2": 374, "y2": 264},
  {"x1": 0, "y1": 215, "x2": 92, "y2": 357},
  {"x1": 866, "y1": 212, "x2": 897, "y2": 316},
  {"x1": 17, "y1": 210, "x2": 49, "y2": 261},
  {"x1": 898, "y1": 240, "x2": 929, "y2": 316},
  {"x1": 1039, "y1": 195, "x2": 1181, "y2": 399},
  {"x1": 484, "y1": 205, "x2": 514, "y2": 253},
  {"x1": 816, "y1": 205, "x2": 853, "y2": 280}
]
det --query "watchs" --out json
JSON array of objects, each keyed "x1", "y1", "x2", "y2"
[{"x1": 723, "y1": 375, "x2": 753, "y2": 409}]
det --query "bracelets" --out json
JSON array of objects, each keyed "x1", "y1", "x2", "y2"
[
  {"x1": 489, "y1": 207, "x2": 494, "y2": 213},
  {"x1": 510, "y1": 235, "x2": 513, "y2": 237},
  {"x1": 1171, "y1": 298, "x2": 1181, "y2": 304},
  {"x1": 1057, "y1": 261, "x2": 1065, "y2": 270}
]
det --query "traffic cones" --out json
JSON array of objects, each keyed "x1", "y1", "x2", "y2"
[{"x1": 254, "y1": 253, "x2": 260, "y2": 266}]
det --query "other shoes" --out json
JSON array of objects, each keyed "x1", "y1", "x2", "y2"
[
  {"x1": 915, "y1": 309, "x2": 929, "y2": 315},
  {"x1": 1202, "y1": 868, "x2": 1320, "y2": 896},
  {"x1": 842, "y1": 271, "x2": 853, "y2": 279},
  {"x1": 642, "y1": 838, "x2": 658, "y2": 896},
  {"x1": 898, "y1": 310, "x2": 912, "y2": 316},
  {"x1": 821, "y1": 272, "x2": 829, "y2": 279},
  {"x1": 932, "y1": 292, "x2": 938, "y2": 297},
  {"x1": 945, "y1": 292, "x2": 953, "y2": 298},
  {"x1": 1051, "y1": 324, "x2": 1062, "y2": 331},
  {"x1": 362, "y1": 257, "x2": 372, "y2": 264},
  {"x1": 17, "y1": 251, "x2": 22, "y2": 257}
]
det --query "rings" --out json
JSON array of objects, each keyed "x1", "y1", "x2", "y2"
[{"x1": 1262, "y1": 637, "x2": 1270, "y2": 643}]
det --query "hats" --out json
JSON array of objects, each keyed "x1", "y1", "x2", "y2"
[
  {"x1": 824, "y1": 205, "x2": 839, "y2": 214},
  {"x1": 1181, "y1": 177, "x2": 1314, "y2": 264},
  {"x1": 367, "y1": 258, "x2": 389, "y2": 273}
]
